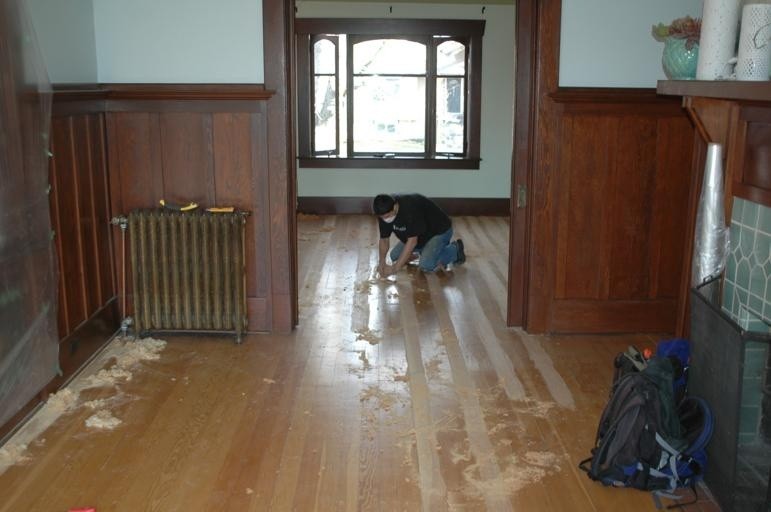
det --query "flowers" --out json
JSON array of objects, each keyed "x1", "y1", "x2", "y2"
[{"x1": 650, "y1": 13, "x2": 702, "y2": 48}]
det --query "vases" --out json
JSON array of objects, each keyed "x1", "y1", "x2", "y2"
[{"x1": 662, "y1": 38, "x2": 699, "y2": 80}]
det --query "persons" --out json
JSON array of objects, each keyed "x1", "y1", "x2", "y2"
[{"x1": 373, "y1": 194, "x2": 465, "y2": 278}]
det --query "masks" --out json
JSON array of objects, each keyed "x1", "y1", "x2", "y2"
[{"x1": 382, "y1": 214, "x2": 396, "y2": 224}]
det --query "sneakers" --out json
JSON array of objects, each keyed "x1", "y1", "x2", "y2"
[{"x1": 452, "y1": 239, "x2": 465, "y2": 265}]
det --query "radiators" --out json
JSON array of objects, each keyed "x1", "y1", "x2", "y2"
[{"x1": 111, "y1": 203, "x2": 256, "y2": 345}]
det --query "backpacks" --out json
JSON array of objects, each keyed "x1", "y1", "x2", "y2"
[{"x1": 589, "y1": 344, "x2": 716, "y2": 490}]
